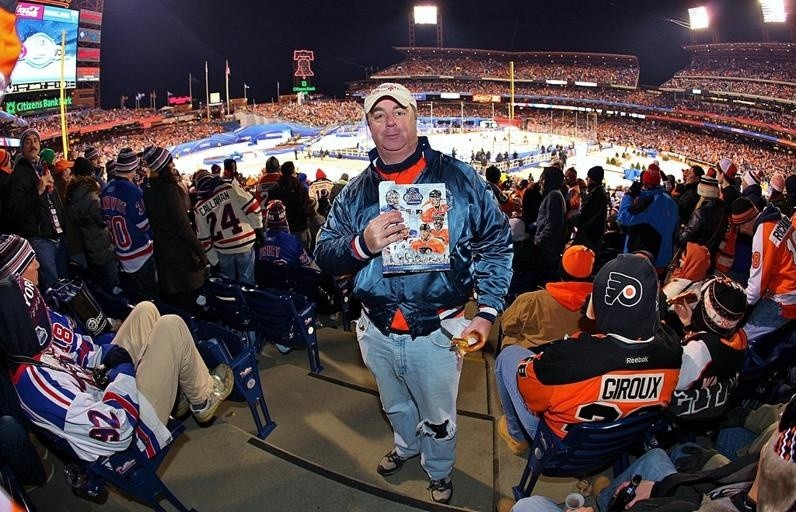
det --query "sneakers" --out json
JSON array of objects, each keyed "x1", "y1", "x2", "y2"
[
  {"x1": 496, "y1": 497, "x2": 516, "y2": 512},
  {"x1": 426, "y1": 476, "x2": 454, "y2": 504},
  {"x1": 188, "y1": 363, "x2": 235, "y2": 423},
  {"x1": 173, "y1": 396, "x2": 191, "y2": 418},
  {"x1": 376, "y1": 447, "x2": 408, "y2": 477},
  {"x1": 14, "y1": 447, "x2": 56, "y2": 495},
  {"x1": 592, "y1": 475, "x2": 612, "y2": 500},
  {"x1": 497, "y1": 413, "x2": 530, "y2": 456}
]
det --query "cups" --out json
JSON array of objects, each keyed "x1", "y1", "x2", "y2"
[{"x1": 564, "y1": 492, "x2": 585, "y2": 508}]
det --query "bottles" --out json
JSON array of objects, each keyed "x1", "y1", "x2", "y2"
[
  {"x1": 43, "y1": 160, "x2": 53, "y2": 193},
  {"x1": 196, "y1": 295, "x2": 210, "y2": 313},
  {"x1": 606, "y1": 472, "x2": 643, "y2": 510}
]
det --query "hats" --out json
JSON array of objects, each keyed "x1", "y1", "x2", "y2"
[
  {"x1": 19, "y1": 127, "x2": 41, "y2": 157},
  {"x1": 264, "y1": 199, "x2": 289, "y2": 227},
  {"x1": 266, "y1": 156, "x2": 280, "y2": 172},
  {"x1": 363, "y1": 82, "x2": 418, "y2": 120},
  {"x1": 587, "y1": 166, "x2": 605, "y2": 182},
  {"x1": 114, "y1": 147, "x2": 141, "y2": 174},
  {"x1": 591, "y1": 252, "x2": 660, "y2": 337},
  {"x1": 316, "y1": 168, "x2": 326, "y2": 179},
  {"x1": 0, "y1": 233, "x2": 36, "y2": 280},
  {"x1": 142, "y1": 144, "x2": 172, "y2": 173},
  {"x1": 194, "y1": 169, "x2": 215, "y2": 189},
  {"x1": 696, "y1": 157, "x2": 785, "y2": 193},
  {"x1": 730, "y1": 196, "x2": 760, "y2": 224},
  {"x1": 557, "y1": 244, "x2": 596, "y2": 282},
  {"x1": 641, "y1": 161, "x2": 661, "y2": 186},
  {"x1": 701, "y1": 276, "x2": 749, "y2": 339},
  {"x1": 0, "y1": 146, "x2": 117, "y2": 177}
]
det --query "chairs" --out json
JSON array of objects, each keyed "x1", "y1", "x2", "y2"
[
  {"x1": 0, "y1": 212, "x2": 358, "y2": 512},
  {"x1": 492, "y1": 230, "x2": 796, "y2": 500}
]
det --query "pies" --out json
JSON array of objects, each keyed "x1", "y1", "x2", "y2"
[{"x1": 665, "y1": 290, "x2": 699, "y2": 305}]
